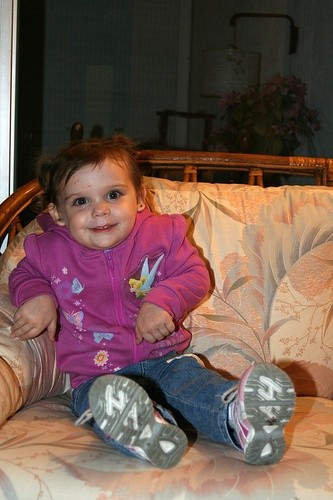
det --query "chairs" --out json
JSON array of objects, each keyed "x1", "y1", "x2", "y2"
[{"x1": 0, "y1": 145, "x2": 333, "y2": 499}]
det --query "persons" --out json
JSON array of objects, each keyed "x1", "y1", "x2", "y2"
[{"x1": 10, "y1": 134, "x2": 296, "y2": 469}]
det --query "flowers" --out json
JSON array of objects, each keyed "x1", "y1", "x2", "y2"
[{"x1": 208, "y1": 73, "x2": 323, "y2": 156}]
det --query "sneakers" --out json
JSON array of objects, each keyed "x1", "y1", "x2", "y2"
[
  {"x1": 74, "y1": 374, "x2": 188, "y2": 471},
  {"x1": 219, "y1": 362, "x2": 296, "y2": 466}
]
